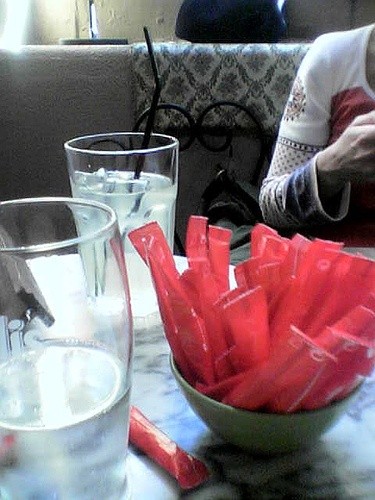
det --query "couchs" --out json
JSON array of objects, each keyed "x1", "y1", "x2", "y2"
[{"x1": 0, "y1": 44, "x2": 315, "y2": 257}]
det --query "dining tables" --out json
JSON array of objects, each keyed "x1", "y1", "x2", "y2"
[{"x1": 0, "y1": 252, "x2": 375, "y2": 500}]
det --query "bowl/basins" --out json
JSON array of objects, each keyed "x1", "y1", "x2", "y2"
[{"x1": 170, "y1": 352, "x2": 364, "y2": 452}]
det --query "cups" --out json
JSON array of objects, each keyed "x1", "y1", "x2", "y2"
[
  {"x1": 1, "y1": 197, "x2": 134, "y2": 500},
  {"x1": 65, "y1": 132, "x2": 178, "y2": 327}
]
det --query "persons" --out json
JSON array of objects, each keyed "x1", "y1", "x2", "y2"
[{"x1": 260, "y1": 22, "x2": 375, "y2": 250}]
